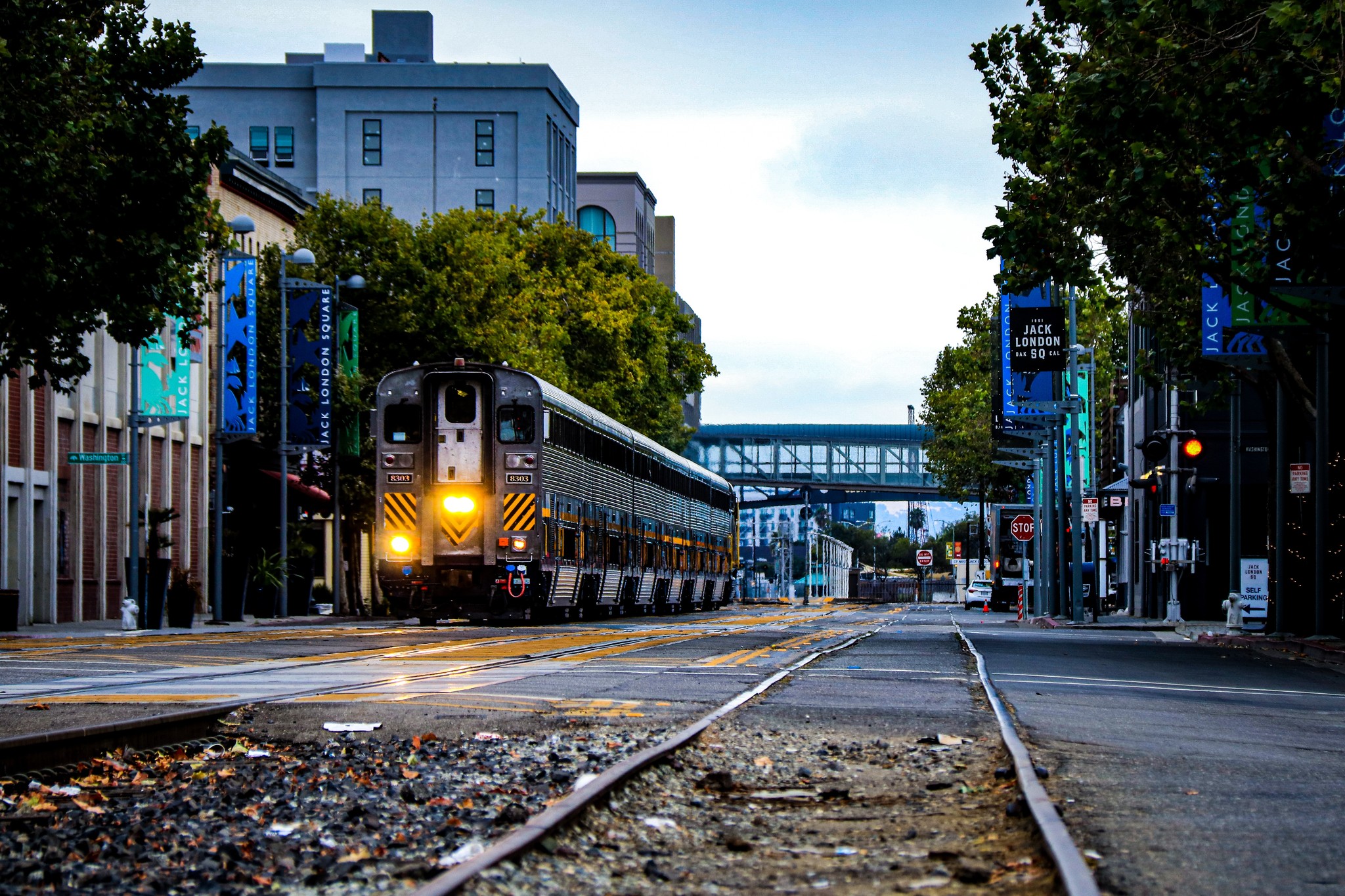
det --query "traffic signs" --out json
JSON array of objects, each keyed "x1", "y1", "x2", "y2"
[{"x1": 955, "y1": 564, "x2": 957, "y2": 567}]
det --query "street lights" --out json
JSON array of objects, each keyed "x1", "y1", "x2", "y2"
[
  {"x1": 807, "y1": 532, "x2": 853, "y2": 598},
  {"x1": 204, "y1": 215, "x2": 259, "y2": 625},
  {"x1": 331, "y1": 272, "x2": 370, "y2": 617},
  {"x1": 270, "y1": 246, "x2": 319, "y2": 612},
  {"x1": 1064, "y1": 342, "x2": 1102, "y2": 626}
]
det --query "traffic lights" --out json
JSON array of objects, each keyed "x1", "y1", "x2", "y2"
[
  {"x1": 1144, "y1": 430, "x2": 1203, "y2": 462},
  {"x1": 1131, "y1": 473, "x2": 1163, "y2": 494}
]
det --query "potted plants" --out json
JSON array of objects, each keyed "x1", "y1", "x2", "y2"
[
  {"x1": 279, "y1": 519, "x2": 320, "y2": 616},
  {"x1": 209, "y1": 525, "x2": 250, "y2": 620},
  {"x1": 166, "y1": 565, "x2": 202, "y2": 629},
  {"x1": 248, "y1": 546, "x2": 305, "y2": 621},
  {"x1": 123, "y1": 504, "x2": 181, "y2": 630}
]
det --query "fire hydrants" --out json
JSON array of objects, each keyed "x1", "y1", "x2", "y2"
[{"x1": 1221, "y1": 591, "x2": 1246, "y2": 637}]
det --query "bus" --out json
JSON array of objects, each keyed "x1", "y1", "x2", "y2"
[{"x1": 372, "y1": 356, "x2": 741, "y2": 619}]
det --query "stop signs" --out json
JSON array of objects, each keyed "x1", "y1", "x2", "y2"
[
  {"x1": 1011, "y1": 514, "x2": 1034, "y2": 541},
  {"x1": 916, "y1": 549, "x2": 933, "y2": 566}
]
